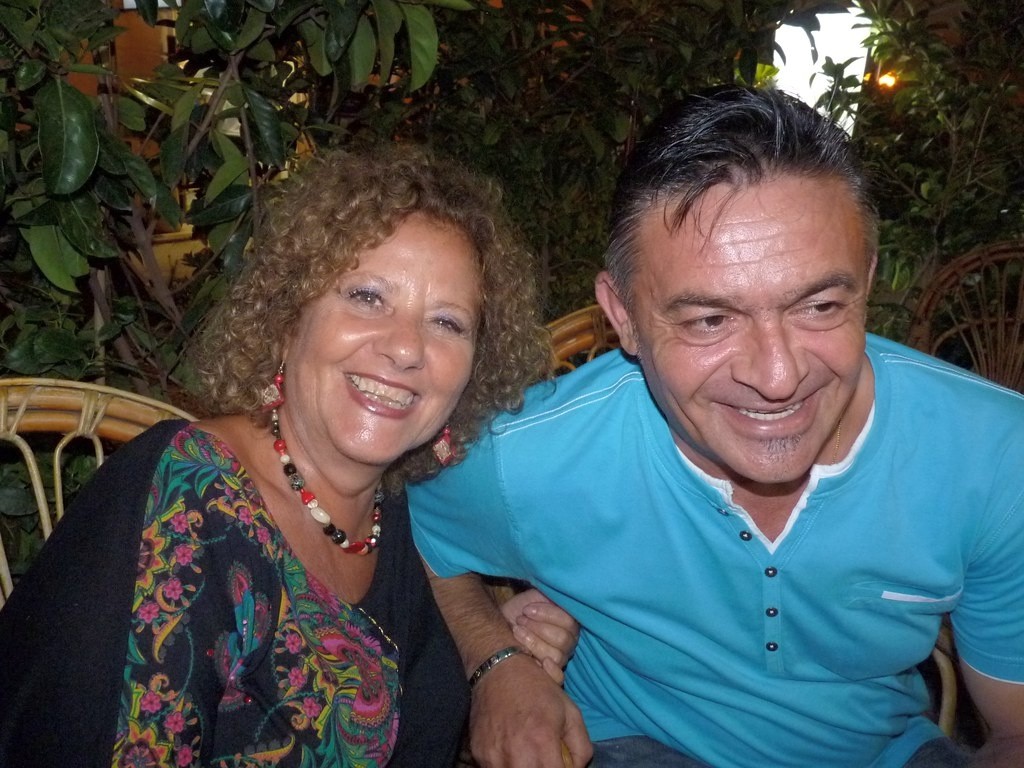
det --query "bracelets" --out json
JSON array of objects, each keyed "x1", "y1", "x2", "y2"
[{"x1": 469, "y1": 646, "x2": 535, "y2": 689}]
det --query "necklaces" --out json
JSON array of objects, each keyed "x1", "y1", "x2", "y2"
[{"x1": 271, "y1": 407, "x2": 385, "y2": 555}]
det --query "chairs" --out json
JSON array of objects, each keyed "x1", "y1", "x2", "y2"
[
  {"x1": 0, "y1": 377, "x2": 201, "y2": 610},
  {"x1": 477, "y1": 303, "x2": 993, "y2": 768},
  {"x1": 906, "y1": 238, "x2": 1024, "y2": 395}
]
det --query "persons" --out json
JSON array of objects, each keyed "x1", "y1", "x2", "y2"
[
  {"x1": 404, "y1": 84, "x2": 1023, "y2": 768},
  {"x1": 0, "y1": 146, "x2": 559, "y2": 768}
]
